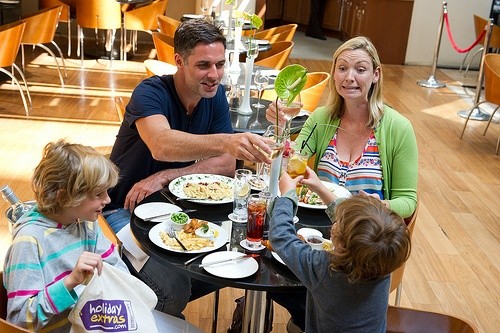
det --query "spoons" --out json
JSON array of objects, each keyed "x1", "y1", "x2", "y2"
[{"x1": 176, "y1": 192, "x2": 227, "y2": 201}]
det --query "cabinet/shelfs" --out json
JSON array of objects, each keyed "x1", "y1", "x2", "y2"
[{"x1": 266, "y1": 0, "x2": 368, "y2": 41}]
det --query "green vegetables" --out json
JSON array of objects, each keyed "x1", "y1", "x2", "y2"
[
  {"x1": 299, "y1": 183, "x2": 318, "y2": 204},
  {"x1": 200, "y1": 223, "x2": 209, "y2": 232},
  {"x1": 171, "y1": 213, "x2": 188, "y2": 224}
]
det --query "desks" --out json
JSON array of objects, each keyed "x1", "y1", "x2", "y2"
[{"x1": 132, "y1": 13, "x2": 333, "y2": 333}]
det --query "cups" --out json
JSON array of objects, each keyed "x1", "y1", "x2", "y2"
[
  {"x1": 259, "y1": 192, "x2": 276, "y2": 237},
  {"x1": 297, "y1": 228, "x2": 325, "y2": 250},
  {"x1": 232, "y1": 170, "x2": 253, "y2": 222},
  {"x1": 283, "y1": 149, "x2": 310, "y2": 198},
  {"x1": 168, "y1": 212, "x2": 189, "y2": 230},
  {"x1": 247, "y1": 193, "x2": 267, "y2": 249},
  {"x1": 194, "y1": 228, "x2": 214, "y2": 243}
]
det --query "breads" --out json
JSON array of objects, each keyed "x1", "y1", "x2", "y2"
[{"x1": 260, "y1": 234, "x2": 307, "y2": 252}]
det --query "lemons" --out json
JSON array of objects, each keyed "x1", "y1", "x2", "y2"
[
  {"x1": 237, "y1": 185, "x2": 249, "y2": 197},
  {"x1": 294, "y1": 160, "x2": 307, "y2": 174}
]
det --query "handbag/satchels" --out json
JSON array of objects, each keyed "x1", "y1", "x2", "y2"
[{"x1": 67, "y1": 262, "x2": 159, "y2": 333}]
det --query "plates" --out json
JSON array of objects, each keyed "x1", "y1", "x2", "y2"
[
  {"x1": 134, "y1": 202, "x2": 183, "y2": 223},
  {"x1": 277, "y1": 180, "x2": 352, "y2": 209},
  {"x1": 148, "y1": 220, "x2": 229, "y2": 253},
  {"x1": 169, "y1": 174, "x2": 279, "y2": 205},
  {"x1": 202, "y1": 239, "x2": 335, "y2": 279}
]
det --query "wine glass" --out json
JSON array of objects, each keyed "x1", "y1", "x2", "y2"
[
  {"x1": 248, "y1": 124, "x2": 288, "y2": 191},
  {"x1": 252, "y1": 68, "x2": 271, "y2": 108}
]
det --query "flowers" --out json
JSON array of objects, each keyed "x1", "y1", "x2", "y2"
[
  {"x1": 236, "y1": 10, "x2": 247, "y2": 27},
  {"x1": 225, "y1": 0, "x2": 237, "y2": 9},
  {"x1": 276, "y1": 64, "x2": 309, "y2": 153},
  {"x1": 243, "y1": 13, "x2": 264, "y2": 60}
]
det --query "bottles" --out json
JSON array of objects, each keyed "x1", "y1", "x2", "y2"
[{"x1": 228, "y1": 83, "x2": 241, "y2": 112}]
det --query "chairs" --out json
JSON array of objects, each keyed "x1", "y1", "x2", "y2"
[
  {"x1": 457, "y1": 14, "x2": 500, "y2": 77},
  {"x1": 285, "y1": 200, "x2": 480, "y2": 333},
  {"x1": 0, "y1": 0, "x2": 331, "y2": 120},
  {"x1": 460, "y1": 53, "x2": 500, "y2": 154}
]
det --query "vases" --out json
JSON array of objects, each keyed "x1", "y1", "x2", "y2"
[
  {"x1": 236, "y1": 58, "x2": 254, "y2": 115},
  {"x1": 268, "y1": 152, "x2": 281, "y2": 197},
  {"x1": 227, "y1": 9, "x2": 232, "y2": 41},
  {"x1": 230, "y1": 25, "x2": 240, "y2": 72}
]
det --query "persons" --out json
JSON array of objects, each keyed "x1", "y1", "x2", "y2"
[
  {"x1": 265, "y1": 37, "x2": 416, "y2": 333},
  {"x1": 102, "y1": 17, "x2": 281, "y2": 324},
  {"x1": 4, "y1": 139, "x2": 200, "y2": 333}
]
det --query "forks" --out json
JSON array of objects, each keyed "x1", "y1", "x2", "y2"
[{"x1": 168, "y1": 230, "x2": 187, "y2": 252}]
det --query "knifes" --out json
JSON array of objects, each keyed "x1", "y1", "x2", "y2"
[
  {"x1": 199, "y1": 254, "x2": 260, "y2": 268},
  {"x1": 143, "y1": 210, "x2": 198, "y2": 222}
]
